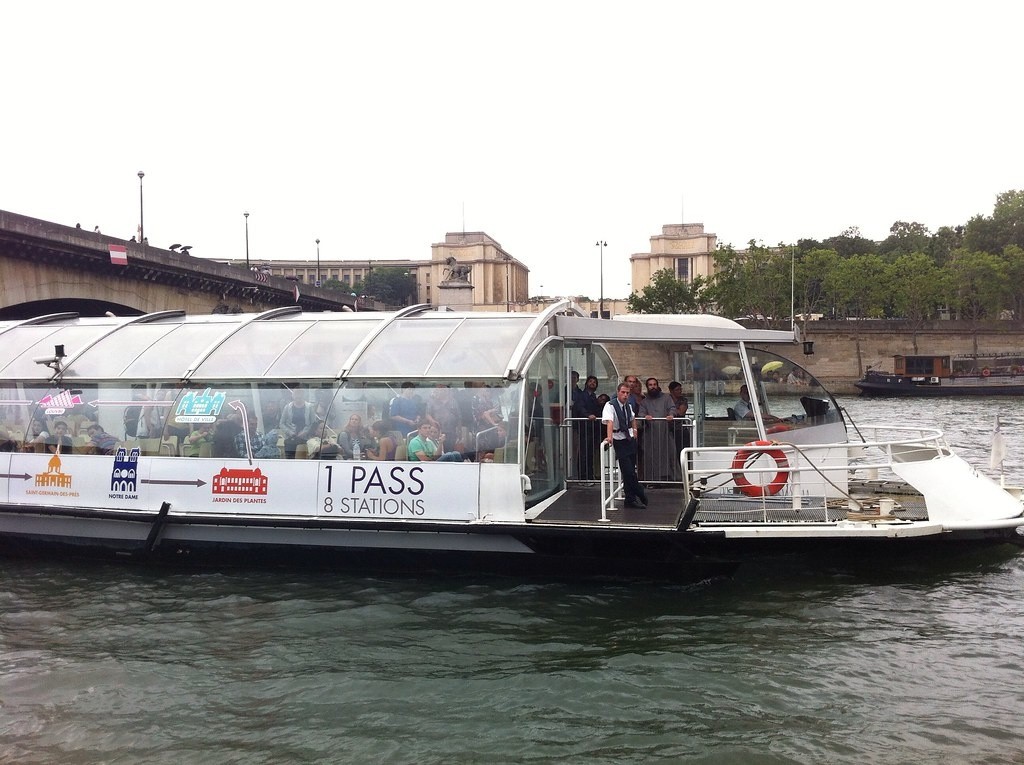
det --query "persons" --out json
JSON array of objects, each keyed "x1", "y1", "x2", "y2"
[
  {"x1": 280, "y1": 381, "x2": 543, "y2": 462},
  {"x1": 554, "y1": 371, "x2": 690, "y2": 488},
  {"x1": 126, "y1": 384, "x2": 279, "y2": 459},
  {"x1": 764, "y1": 367, "x2": 808, "y2": 386},
  {"x1": 602, "y1": 382, "x2": 648, "y2": 508},
  {"x1": 27, "y1": 418, "x2": 120, "y2": 455},
  {"x1": 733, "y1": 385, "x2": 790, "y2": 423}
]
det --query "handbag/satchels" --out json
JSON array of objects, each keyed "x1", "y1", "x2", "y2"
[{"x1": 148, "y1": 426, "x2": 162, "y2": 438}]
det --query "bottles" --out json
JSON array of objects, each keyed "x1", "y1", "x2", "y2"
[{"x1": 353, "y1": 442, "x2": 361, "y2": 460}]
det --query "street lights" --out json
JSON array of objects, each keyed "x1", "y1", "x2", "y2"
[
  {"x1": 502, "y1": 257, "x2": 511, "y2": 312},
  {"x1": 243, "y1": 212, "x2": 250, "y2": 271},
  {"x1": 367, "y1": 259, "x2": 373, "y2": 300},
  {"x1": 316, "y1": 238, "x2": 321, "y2": 287},
  {"x1": 137, "y1": 170, "x2": 145, "y2": 244},
  {"x1": 596, "y1": 238, "x2": 608, "y2": 317}
]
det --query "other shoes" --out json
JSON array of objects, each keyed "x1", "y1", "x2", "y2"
[
  {"x1": 624, "y1": 497, "x2": 647, "y2": 509},
  {"x1": 638, "y1": 485, "x2": 648, "y2": 507},
  {"x1": 577, "y1": 483, "x2": 595, "y2": 486}
]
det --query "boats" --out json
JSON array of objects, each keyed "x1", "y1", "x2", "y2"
[
  {"x1": 0, "y1": 297, "x2": 1024, "y2": 564},
  {"x1": 853, "y1": 349, "x2": 1023, "y2": 398}
]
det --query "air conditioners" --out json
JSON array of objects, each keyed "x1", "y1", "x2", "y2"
[{"x1": 931, "y1": 377, "x2": 939, "y2": 383}]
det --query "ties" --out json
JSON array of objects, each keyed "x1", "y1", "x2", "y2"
[{"x1": 622, "y1": 406, "x2": 626, "y2": 425}]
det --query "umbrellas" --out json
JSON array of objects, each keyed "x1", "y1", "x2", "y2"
[
  {"x1": 761, "y1": 361, "x2": 783, "y2": 375},
  {"x1": 721, "y1": 366, "x2": 741, "y2": 376}
]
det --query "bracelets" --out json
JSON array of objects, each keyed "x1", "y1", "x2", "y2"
[{"x1": 633, "y1": 428, "x2": 638, "y2": 431}]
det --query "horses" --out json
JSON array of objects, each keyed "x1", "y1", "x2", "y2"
[{"x1": 443, "y1": 257, "x2": 472, "y2": 283}]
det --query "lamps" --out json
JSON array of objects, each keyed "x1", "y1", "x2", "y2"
[
  {"x1": 704, "y1": 342, "x2": 715, "y2": 351},
  {"x1": 55, "y1": 345, "x2": 66, "y2": 357}
]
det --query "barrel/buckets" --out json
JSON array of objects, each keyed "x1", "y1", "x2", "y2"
[
  {"x1": 849, "y1": 500, "x2": 862, "y2": 512},
  {"x1": 879, "y1": 500, "x2": 894, "y2": 516}
]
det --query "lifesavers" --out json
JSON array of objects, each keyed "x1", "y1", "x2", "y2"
[
  {"x1": 982, "y1": 369, "x2": 990, "y2": 377},
  {"x1": 767, "y1": 424, "x2": 790, "y2": 435},
  {"x1": 732, "y1": 441, "x2": 790, "y2": 498}
]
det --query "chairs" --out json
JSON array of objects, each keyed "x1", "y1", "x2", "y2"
[
  {"x1": 726, "y1": 408, "x2": 737, "y2": 420},
  {"x1": 4, "y1": 434, "x2": 504, "y2": 465}
]
